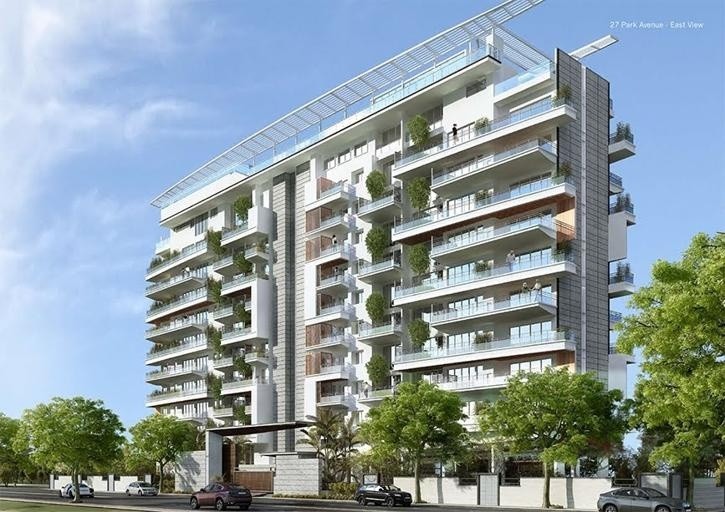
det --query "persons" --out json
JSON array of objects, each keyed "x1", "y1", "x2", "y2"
[
  {"x1": 506, "y1": 249, "x2": 516, "y2": 271},
  {"x1": 533, "y1": 278, "x2": 543, "y2": 302},
  {"x1": 452, "y1": 123, "x2": 460, "y2": 145},
  {"x1": 332, "y1": 233, "x2": 338, "y2": 248},
  {"x1": 522, "y1": 282, "x2": 530, "y2": 301}
]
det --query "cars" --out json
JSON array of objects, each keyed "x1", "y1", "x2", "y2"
[
  {"x1": 126, "y1": 481, "x2": 158, "y2": 496},
  {"x1": 597, "y1": 488, "x2": 684, "y2": 512},
  {"x1": 190, "y1": 482, "x2": 252, "y2": 511},
  {"x1": 356, "y1": 484, "x2": 412, "y2": 507},
  {"x1": 60, "y1": 483, "x2": 94, "y2": 498}
]
